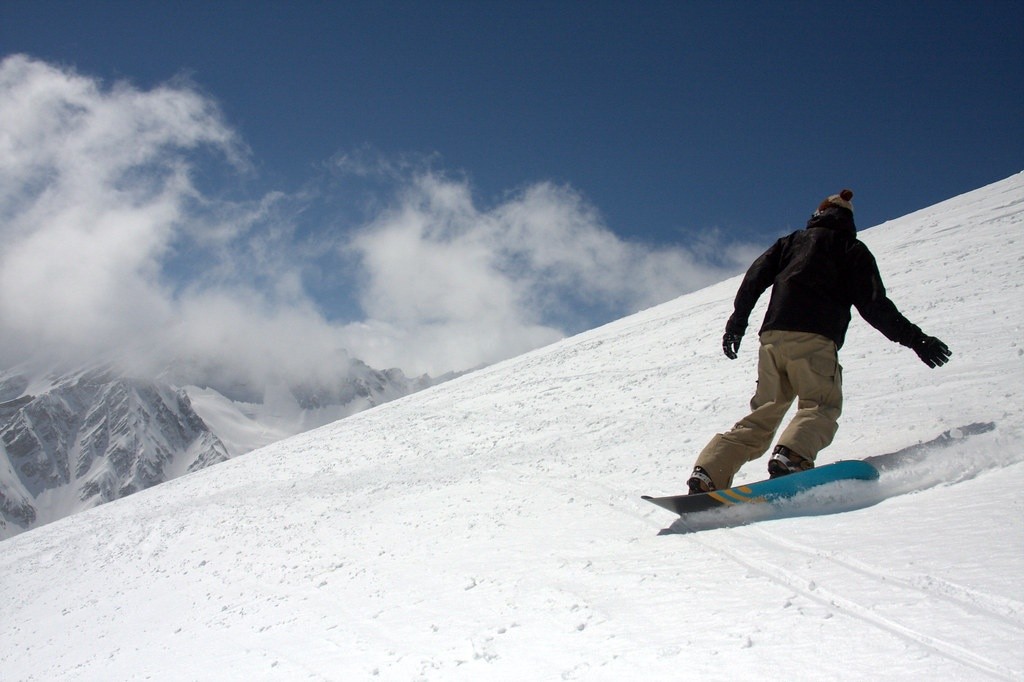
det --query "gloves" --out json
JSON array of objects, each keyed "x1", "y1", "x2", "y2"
[
  {"x1": 910, "y1": 332, "x2": 952, "y2": 369},
  {"x1": 722, "y1": 332, "x2": 742, "y2": 359}
]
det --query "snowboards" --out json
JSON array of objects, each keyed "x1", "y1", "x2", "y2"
[{"x1": 640, "y1": 460, "x2": 879, "y2": 515}]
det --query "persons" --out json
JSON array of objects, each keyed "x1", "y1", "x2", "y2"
[{"x1": 686, "y1": 190, "x2": 951, "y2": 495}]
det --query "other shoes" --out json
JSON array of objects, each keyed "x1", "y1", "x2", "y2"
[
  {"x1": 767, "y1": 445, "x2": 814, "y2": 478},
  {"x1": 687, "y1": 466, "x2": 716, "y2": 495}
]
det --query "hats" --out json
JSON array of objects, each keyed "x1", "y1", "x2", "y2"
[{"x1": 813, "y1": 189, "x2": 853, "y2": 217}]
